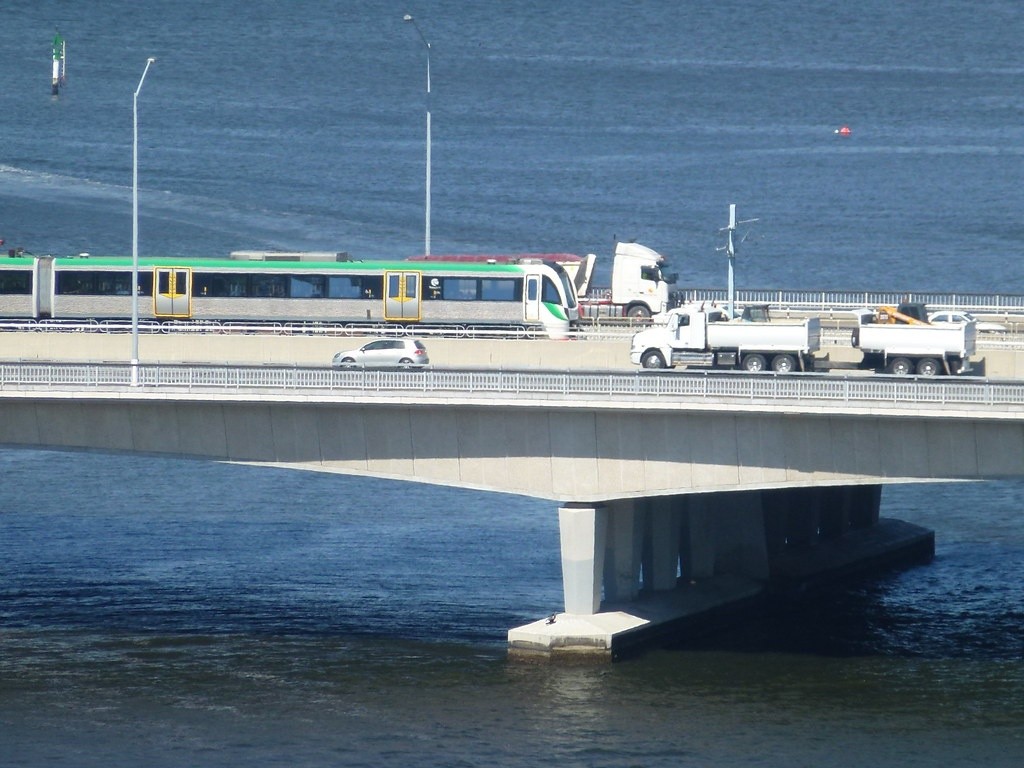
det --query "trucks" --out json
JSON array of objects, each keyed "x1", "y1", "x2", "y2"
[{"x1": 629, "y1": 305, "x2": 983, "y2": 377}]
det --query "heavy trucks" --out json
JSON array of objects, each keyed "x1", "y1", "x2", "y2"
[{"x1": 406, "y1": 236, "x2": 687, "y2": 328}]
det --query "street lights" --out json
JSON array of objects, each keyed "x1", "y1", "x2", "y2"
[
  {"x1": 131, "y1": 55, "x2": 159, "y2": 386},
  {"x1": 403, "y1": 14, "x2": 432, "y2": 256}
]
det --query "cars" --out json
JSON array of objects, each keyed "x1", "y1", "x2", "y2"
[{"x1": 928, "y1": 309, "x2": 1007, "y2": 336}]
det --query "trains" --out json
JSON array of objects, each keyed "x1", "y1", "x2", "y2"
[{"x1": 0, "y1": 249, "x2": 587, "y2": 341}]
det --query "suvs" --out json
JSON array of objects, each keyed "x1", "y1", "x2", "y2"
[{"x1": 330, "y1": 337, "x2": 431, "y2": 373}]
